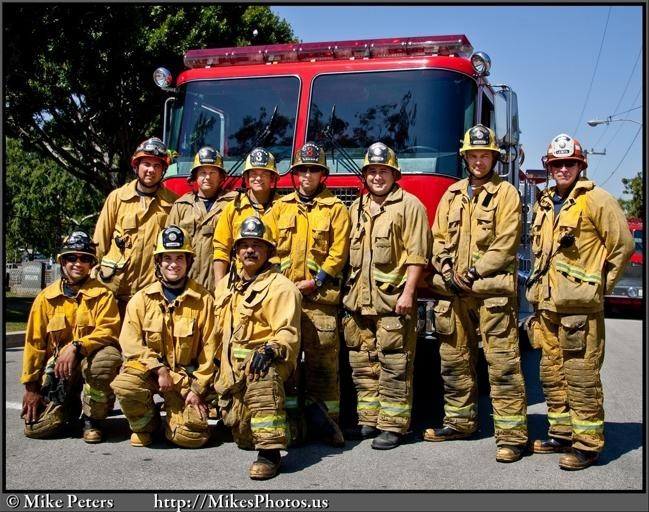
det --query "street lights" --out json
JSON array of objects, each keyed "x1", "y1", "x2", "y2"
[{"x1": 587, "y1": 116, "x2": 642, "y2": 128}]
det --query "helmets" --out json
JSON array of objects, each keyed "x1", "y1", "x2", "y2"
[
  {"x1": 460, "y1": 124, "x2": 499, "y2": 159},
  {"x1": 190, "y1": 147, "x2": 227, "y2": 181},
  {"x1": 233, "y1": 216, "x2": 276, "y2": 248},
  {"x1": 153, "y1": 224, "x2": 196, "y2": 257},
  {"x1": 542, "y1": 134, "x2": 588, "y2": 170},
  {"x1": 291, "y1": 144, "x2": 329, "y2": 174},
  {"x1": 240, "y1": 147, "x2": 280, "y2": 183},
  {"x1": 130, "y1": 137, "x2": 170, "y2": 174},
  {"x1": 56, "y1": 231, "x2": 98, "y2": 267},
  {"x1": 361, "y1": 142, "x2": 401, "y2": 181}
]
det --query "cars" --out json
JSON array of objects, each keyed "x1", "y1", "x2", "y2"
[{"x1": 7, "y1": 247, "x2": 62, "y2": 292}]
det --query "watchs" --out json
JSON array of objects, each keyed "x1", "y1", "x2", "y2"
[
  {"x1": 313, "y1": 277, "x2": 323, "y2": 288},
  {"x1": 71, "y1": 340, "x2": 81, "y2": 351}
]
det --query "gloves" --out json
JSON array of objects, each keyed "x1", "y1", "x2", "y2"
[
  {"x1": 239, "y1": 343, "x2": 285, "y2": 380},
  {"x1": 443, "y1": 268, "x2": 478, "y2": 298}
]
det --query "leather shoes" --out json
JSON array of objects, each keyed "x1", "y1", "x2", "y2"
[
  {"x1": 83, "y1": 417, "x2": 103, "y2": 442},
  {"x1": 560, "y1": 448, "x2": 598, "y2": 469},
  {"x1": 529, "y1": 437, "x2": 572, "y2": 454},
  {"x1": 496, "y1": 445, "x2": 522, "y2": 462},
  {"x1": 131, "y1": 414, "x2": 161, "y2": 447},
  {"x1": 372, "y1": 431, "x2": 401, "y2": 449},
  {"x1": 422, "y1": 427, "x2": 473, "y2": 442},
  {"x1": 250, "y1": 449, "x2": 281, "y2": 478},
  {"x1": 342, "y1": 425, "x2": 380, "y2": 440}
]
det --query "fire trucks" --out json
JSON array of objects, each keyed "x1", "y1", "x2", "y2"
[
  {"x1": 605, "y1": 219, "x2": 644, "y2": 320},
  {"x1": 153, "y1": 35, "x2": 550, "y2": 396}
]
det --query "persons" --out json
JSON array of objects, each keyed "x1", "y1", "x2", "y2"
[
  {"x1": 340, "y1": 142, "x2": 433, "y2": 448},
  {"x1": 524, "y1": 134, "x2": 636, "y2": 471},
  {"x1": 212, "y1": 147, "x2": 287, "y2": 288},
  {"x1": 214, "y1": 216, "x2": 344, "y2": 479},
  {"x1": 164, "y1": 147, "x2": 239, "y2": 298},
  {"x1": 419, "y1": 124, "x2": 530, "y2": 462},
  {"x1": 93, "y1": 138, "x2": 180, "y2": 329},
  {"x1": 259, "y1": 141, "x2": 352, "y2": 446},
  {"x1": 109, "y1": 225, "x2": 215, "y2": 448},
  {"x1": 20, "y1": 231, "x2": 123, "y2": 443}
]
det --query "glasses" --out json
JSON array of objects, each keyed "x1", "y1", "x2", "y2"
[
  {"x1": 142, "y1": 142, "x2": 167, "y2": 155},
  {"x1": 63, "y1": 254, "x2": 92, "y2": 263},
  {"x1": 551, "y1": 160, "x2": 576, "y2": 167},
  {"x1": 296, "y1": 166, "x2": 321, "y2": 172}
]
